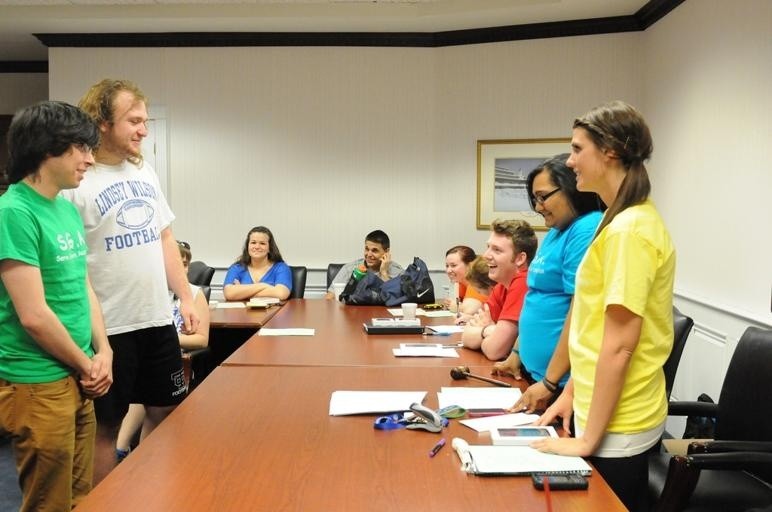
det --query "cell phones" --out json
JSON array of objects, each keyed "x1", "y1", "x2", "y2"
[
  {"x1": 532, "y1": 474, "x2": 589, "y2": 491},
  {"x1": 469, "y1": 408, "x2": 505, "y2": 417}
]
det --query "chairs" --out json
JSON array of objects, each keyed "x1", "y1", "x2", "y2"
[
  {"x1": 171, "y1": 258, "x2": 216, "y2": 397},
  {"x1": 645, "y1": 302, "x2": 695, "y2": 454},
  {"x1": 639, "y1": 323, "x2": 772, "y2": 511},
  {"x1": 324, "y1": 263, "x2": 347, "y2": 290},
  {"x1": 286, "y1": 263, "x2": 309, "y2": 299}
]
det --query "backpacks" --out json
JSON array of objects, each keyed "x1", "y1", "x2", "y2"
[{"x1": 340, "y1": 253, "x2": 437, "y2": 306}]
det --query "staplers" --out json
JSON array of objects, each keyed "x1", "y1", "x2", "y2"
[{"x1": 405, "y1": 402, "x2": 443, "y2": 433}]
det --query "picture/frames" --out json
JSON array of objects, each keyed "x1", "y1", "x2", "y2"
[{"x1": 474, "y1": 134, "x2": 577, "y2": 233}]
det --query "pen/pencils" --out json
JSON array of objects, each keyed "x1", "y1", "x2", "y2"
[
  {"x1": 456, "y1": 297, "x2": 459, "y2": 327},
  {"x1": 467, "y1": 412, "x2": 505, "y2": 418}
]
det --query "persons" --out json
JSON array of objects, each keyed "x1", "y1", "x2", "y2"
[
  {"x1": 532, "y1": 101, "x2": 673, "y2": 511},
  {"x1": 115, "y1": 243, "x2": 210, "y2": 462},
  {"x1": 489, "y1": 152, "x2": 604, "y2": 415},
  {"x1": 224, "y1": 226, "x2": 293, "y2": 301},
  {"x1": 55, "y1": 79, "x2": 200, "y2": 443},
  {"x1": 324, "y1": 230, "x2": 409, "y2": 301},
  {"x1": 462, "y1": 220, "x2": 538, "y2": 360},
  {"x1": 446, "y1": 245, "x2": 476, "y2": 312},
  {"x1": 1, "y1": 101, "x2": 116, "y2": 512},
  {"x1": 454, "y1": 256, "x2": 500, "y2": 325}
]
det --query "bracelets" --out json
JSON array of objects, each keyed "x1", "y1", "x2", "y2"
[
  {"x1": 542, "y1": 379, "x2": 556, "y2": 393},
  {"x1": 480, "y1": 324, "x2": 487, "y2": 338},
  {"x1": 545, "y1": 377, "x2": 558, "y2": 387},
  {"x1": 511, "y1": 349, "x2": 519, "y2": 355}
]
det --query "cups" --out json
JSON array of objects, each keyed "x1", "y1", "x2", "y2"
[{"x1": 401, "y1": 302, "x2": 419, "y2": 320}]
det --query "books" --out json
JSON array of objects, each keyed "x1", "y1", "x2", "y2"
[{"x1": 361, "y1": 320, "x2": 424, "y2": 335}]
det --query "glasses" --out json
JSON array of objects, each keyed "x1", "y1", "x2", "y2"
[{"x1": 533, "y1": 188, "x2": 561, "y2": 204}]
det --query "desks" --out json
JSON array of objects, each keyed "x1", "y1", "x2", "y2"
[
  {"x1": 194, "y1": 293, "x2": 291, "y2": 372},
  {"x1": 219, "y1": 293, "x2": 524, "y2": 373},
  {"x1": 69, "y1": 362, "x2": 630, "y2": 512}
]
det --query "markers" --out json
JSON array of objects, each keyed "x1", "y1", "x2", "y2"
[{"x1": 428, "y1": 439, "x2": 446, "y2": 456}]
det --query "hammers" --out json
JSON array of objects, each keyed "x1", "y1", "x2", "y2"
[{"x1": 450, "y1": 366, "x2": 512, "y2": 387}]
